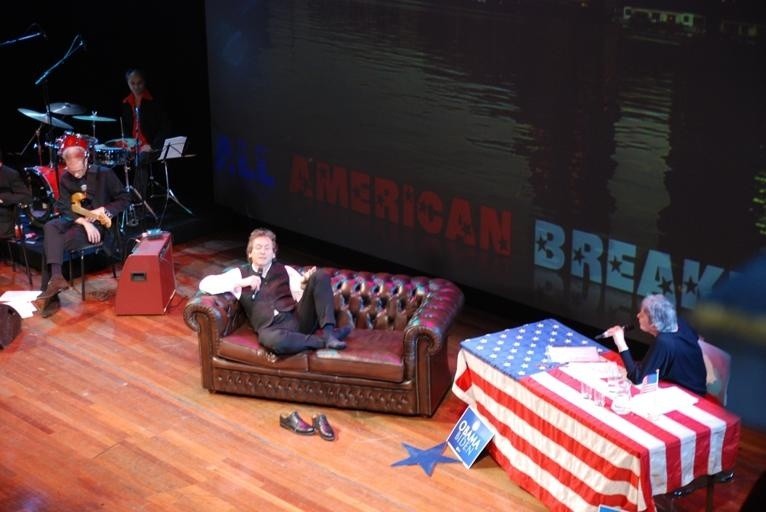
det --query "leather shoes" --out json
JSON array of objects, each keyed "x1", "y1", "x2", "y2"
[
  {"x1": 40, "y1": 296, "x2": 60, "y2": 319},
  {"x1": 279, "y1": 411, "x2": 313, "y2": 436},
  {"x1": 312, "y1": 413, "x2": 335, "y2": 442},
  {"x1": 38, "y1": 277, "x2": 70, "y2": 300}
]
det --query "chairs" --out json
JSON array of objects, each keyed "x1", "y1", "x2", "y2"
[
  {"x1": 41, "y1": 214, "x2": 120, "y2": 301},
  {"x1": 0, "y1": 204, "x2": 34, "y2": 285},
  {"x1": 673, "y1": 337, "x2": 735, "y2": 497}
]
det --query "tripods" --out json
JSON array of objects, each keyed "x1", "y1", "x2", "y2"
[{"x1": 36, "y1": 123, "x2": 193, "y2": 233}]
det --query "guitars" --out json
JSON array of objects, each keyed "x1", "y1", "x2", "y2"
[{"x1": 71, "y1": 193, "x2": 112, "y2": 228}]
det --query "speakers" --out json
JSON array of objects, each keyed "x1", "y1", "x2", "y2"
[
  {"x1": 0, "y1": 304, "x2": 21, "y2": 349},
  {"x1": 115, "y1": 231, "x2": 177, "y2": 316}
]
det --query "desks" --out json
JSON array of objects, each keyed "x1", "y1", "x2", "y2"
[{"x1": 451, "y1": 319, "x2": 741, "y2": 512}]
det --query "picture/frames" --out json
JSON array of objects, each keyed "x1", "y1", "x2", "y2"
[{"x1": 445, "y1": 405, "x2": 495, "y2": 470}]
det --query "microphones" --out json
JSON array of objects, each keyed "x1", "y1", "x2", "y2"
[
  {"x1": 37, "y1": 18, "x2": 49, "y2": 41},
  {"x1": 251, "y1": 268, "x2": 262, "y2": 300},
  {"x1": 77, "y1": 32, "x2": 88, "y2": 53},
  {"x1": 594, "y1": 321, "x2": 634, "y2": 340}
]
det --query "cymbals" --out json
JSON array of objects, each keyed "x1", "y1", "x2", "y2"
[
  {"x1": 74, "y1": 115, "x2": 116, "y2": 122},
  {"x1": 20, "y1": 108, "x2": 72, "y2": 130},
  {"x1": 49, "y1": 103, "x2": 80, "y2": 115}
]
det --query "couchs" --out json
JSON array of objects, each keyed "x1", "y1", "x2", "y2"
[{"x1": 182, "y1": 261, "x2": 466, "y2": 418}]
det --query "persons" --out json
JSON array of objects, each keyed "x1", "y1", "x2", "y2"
[
  {"x1": 118, "y1": 69, "x2": 161, "y2": 198},
  {"x1": 198, "y1": 227, "x2": 350, "y2": 356},
  {"x1": 31, "y1": 146, "x2": 131, "y2": 316},
  {"x1": 0, "y1": 161, "x2": 32, "y2": 239},
  {"x1": 603, "y1": 294, "x2": 707, "y2": 396}
]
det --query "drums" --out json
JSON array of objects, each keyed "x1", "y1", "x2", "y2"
[
  {"x1": 95, "y1": 148, "x2": 127, "y2": 167},
  {"x1": 57, "y1": 130, "x2": 98, "y2": 160},
  {"x1": 24, "y1": 161, "x2": 67, "y2": 221},
  {"x1": 106, "y1": 139, "x2": 140, "y2": 161}
]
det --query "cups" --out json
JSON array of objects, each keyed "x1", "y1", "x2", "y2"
[{"x1": 580, "y1": 373, "x2": 634, "y2": 415}]
